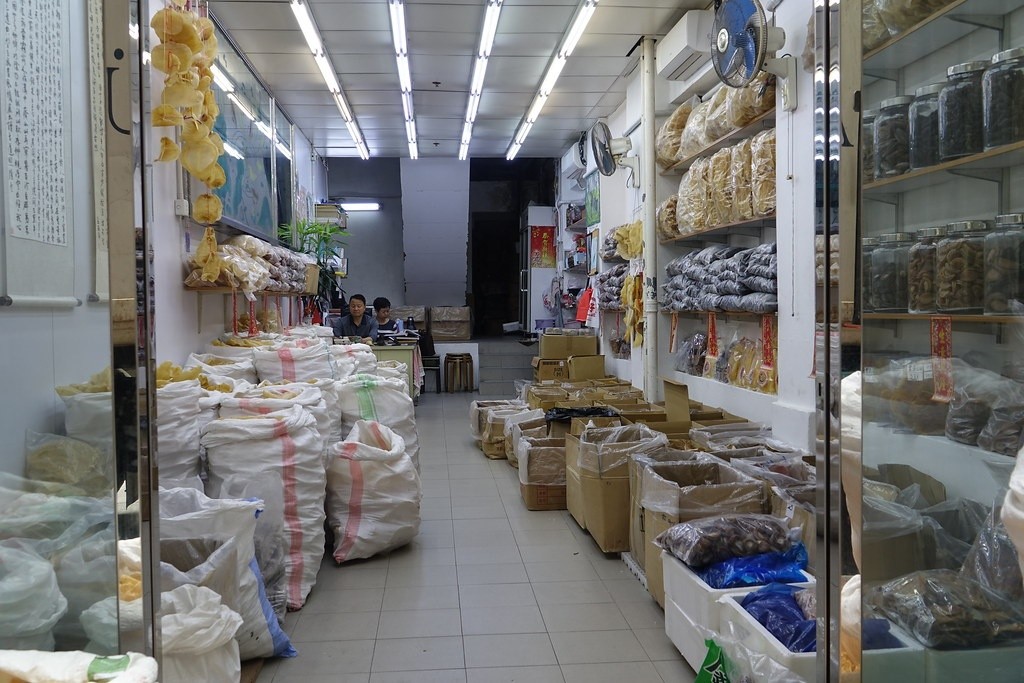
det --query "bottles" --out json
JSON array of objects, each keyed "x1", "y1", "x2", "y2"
[
  {"x1": 907, "y1": 228, "x2": 942, "y2": 315},
  {"x1": 407, "y1": 317, "x2": 417, "y2": 337},
  {"x1": 868, "y1": 233, "x2": 916, "y2": 312},
  {"x1": 984, "y1": 214, "x2": 1024, "y2": 315},
  {"x1": 862, "y1": 238, "x2": 875, "y2": 307},
  {"x1": 936, "y1": 220, "x2": 991, "y2": 313},
  {"x1": 874, "y1": 96, "x2": 923, "y2": 182},
  {"x1": 908, "y1": 82, "x2": 947, "y2": 171},
  {"x1": 862, "y1": 110, "x2": 877, "y2": 184},
  {"x1": 980, "y1": 46, "x2": 1024, "y2": 150},
  {"x1": 937, "y1": 59, "x2": 990, "y2": 161}
]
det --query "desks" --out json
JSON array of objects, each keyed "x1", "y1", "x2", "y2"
[{"x1": 369, "y1": 343, "x2": 418, "y2": 411}]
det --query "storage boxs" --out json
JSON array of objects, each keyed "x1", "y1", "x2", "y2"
[
  {"x1": 471, "y1": 325, "x2": 1024, "y2": 683},
  {"x1": 303, "y1": 261, "x2": 321, "y2": 297},
  {"x1": 389, "y1": 303, "x2": 428, "y2": 336},
  {"x1": 430, "y1": 303, "x2": 471, "y2": 341}
]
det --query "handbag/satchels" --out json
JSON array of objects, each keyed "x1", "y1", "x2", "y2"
[{"x1": 415, "y1": 329, "x2": 435, "y2": 356}]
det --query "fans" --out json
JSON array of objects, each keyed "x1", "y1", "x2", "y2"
[
  {"x1": 590, "y1": 119, "x2": 642, "y2": 189},
  {"x1": 709, "y1": 0, "x2": 800, "y2": 113}
]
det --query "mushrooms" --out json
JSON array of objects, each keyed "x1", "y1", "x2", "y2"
[{"x1": 654, "y1": 515, "x2": 789, "y2": 568}]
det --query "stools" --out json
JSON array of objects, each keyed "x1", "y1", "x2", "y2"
[{"x1": 443, "y1": 352, "x2": 474, "y2": 394}]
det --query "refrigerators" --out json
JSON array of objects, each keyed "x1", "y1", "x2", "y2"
[{"x1": 518, "y1": 204, "x2": 558, "y2": 338}]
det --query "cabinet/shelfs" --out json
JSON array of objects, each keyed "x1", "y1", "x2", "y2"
[
  {"x1": 659, "y1": 16, "x2": 781, "y2": 408},
  {"x1": 864, "y1": 0, "x2": 1024, "y2": 508},
  {"x1": 603, "y1": 255, "x2": 642, "y2": 359},
  {"x1": 565, "y1": 201, "x2": 588, "y2": 277}
]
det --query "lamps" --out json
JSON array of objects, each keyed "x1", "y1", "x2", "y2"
[
  {"x1": 340, "y1": 202, "x2": 384, "y2": 211},
  {"x1": 209, "y1": 59, "x2": 236, "y2": 94},
  {"x1": 275, "y1": 132, "x2": 290, "y2": 161},
  {"x1": 254, "y1": 117, "x2": 279, "y2": 143},
  {"x1": 228, "y1": 88, "x2": 262, "y2": 122},
  {"x1": 287, "y1": 0, "x2": 599, "y2": 161},
  {"x1": 222, "y1": 137, "x2": 245, "y2": 162}
]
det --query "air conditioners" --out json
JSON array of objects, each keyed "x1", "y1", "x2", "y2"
[
  {"x1": 656, "y1": 9, "x2": 716, "y2": 81},
  {"x1": 560, "y1": 140, "x2": 588, "y2": 180}
]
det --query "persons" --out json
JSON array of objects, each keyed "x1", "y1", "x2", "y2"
[
  {"x1": 332, "y1": 292, "x2": 379, "y2": 343},
  {"x1": 372, "y1": 296, "x2": 400, "y2": 346}
]
